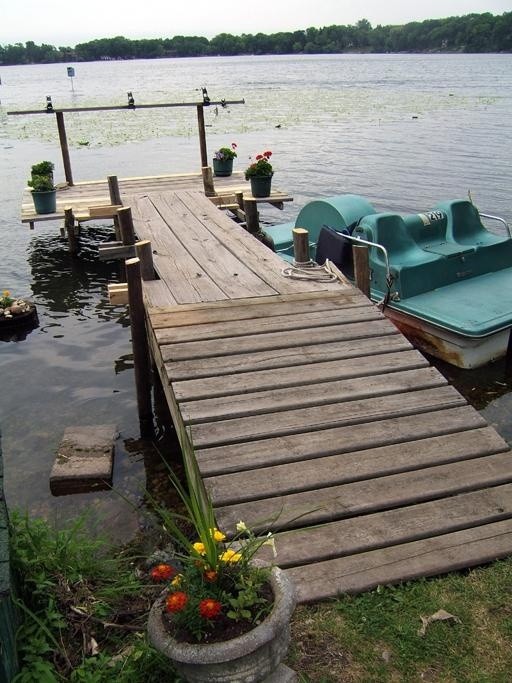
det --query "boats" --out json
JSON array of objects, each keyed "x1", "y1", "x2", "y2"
[{"x1": 263, "y1": 185, "x2": 512, "y2": 375}]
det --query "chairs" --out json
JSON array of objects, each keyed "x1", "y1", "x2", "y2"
[
  {"x1": 435, "y1": 197, "x2": 512, "y2": 273},
  {"x1": 361, "y1": 212, "x2": 443, "y2": 300}
]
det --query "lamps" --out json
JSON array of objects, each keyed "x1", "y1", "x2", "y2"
[{"x1": 45, "y1": 86, "x2": 209, "y2": 112}]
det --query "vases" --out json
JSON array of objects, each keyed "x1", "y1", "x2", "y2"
[
  {"x1": 249, "y1": 173, "x2": 272, "y2": 198},
  {"x1": 145, "y1": 559, "x2": 298, "y2": 683},
  {"x1": 214, "y1": 158, "x2": 233, "y2": 176}
]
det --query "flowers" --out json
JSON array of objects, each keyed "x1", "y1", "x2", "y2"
[
  {"x1": 242, "y1": 150, "x2": 277, "y2": 180},
  {"x1": 214, "y1": 140, "x2": 239, "y2": 161},
  {"x1": 76, "y1": 427, "x2": 336, "y2": 642}
]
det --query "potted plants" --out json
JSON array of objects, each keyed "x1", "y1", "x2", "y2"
[
  {"x1": 27, "y1": 175, "x2": 58, "y2": 213},
  {"x1": 30, "y1": 160, "x2": 55, "y2": 182}
]
